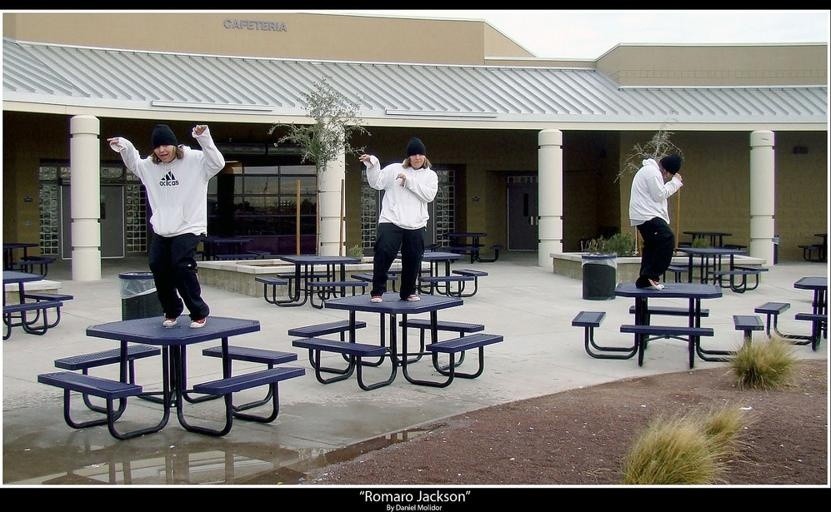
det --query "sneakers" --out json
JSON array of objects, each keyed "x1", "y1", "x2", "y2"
[
  {"x1": 370, "y1": 288, "x2": 384, "y2": 304},
  {"x1": 637, "y1": 279, "x2": 665, "y2": 289},
  {"x1": 647, "y1": 273, "x2": 663, "y2": 291},
  {"x1": 400, "y1": 291, "x2": 420, "y2": 302},
  {"x1": 162, "y1": 303, "x2": 183, "y2": 328},
  {"x1": 190, "y1": 311, "x2": 208, "y2": 329}
]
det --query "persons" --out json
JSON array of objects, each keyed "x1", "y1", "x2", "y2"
[
  {"x1": 631, "y1": 154, "x2": 685, "y2": 291},
  {"x1": 107, "y1": 124, "x2": 226, "y2": 329},
  {"x1": 360, "y1": 137, "x2": 439, "y2": 303}
]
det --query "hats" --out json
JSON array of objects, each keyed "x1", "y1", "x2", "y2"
[
  {"x1": 659, "y1": 153, "x2": 683, "y2": 176},
  {"x1": 406, "y1": 136, "x2": 426, "y2": 157},
  {"x1": 151, "y1": 123, "x2": 178, "y2": 150}
]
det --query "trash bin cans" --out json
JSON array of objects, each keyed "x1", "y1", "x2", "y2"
[
  {"x1": 581, "y1": 252, "x2": 617, "y2": 300},
  {"x1": 118, "y1": 271, "x2": 164, "y2": 322},
  {"x1": 772, "y1": 237, "x2": 779, "y2": 265}
]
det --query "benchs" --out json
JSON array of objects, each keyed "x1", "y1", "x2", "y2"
[
  {"x1": 422, "y1": 241, "x2": 501, "y2": 263},
  {"x1": 575, "y1": 227, "x2": 828, "y2": 373},
  {"x1": 10, "y1": 253, "x2": 55, "y2": 277},
  {"x1": 258, "y1": 266, "x2": 488, "y2": 307},
  {"x1": 38, "y1": 345, "x2": 162, "y2": 440},
  {"x1": 0, "y1": 292, "x2": 73, "y2": 340},
  {"x1": 399, "y1": 318, "x2": 503, "y2": 387},
  {"x1": 287, "y1": 320, "x2": 396, "y2": 391},
  {"x1": 200, "y1": 251, "x2": 269, "y2": 260},
  {"x1": 191, "y1": 344, "x2": 306, "y2": 437}
]
